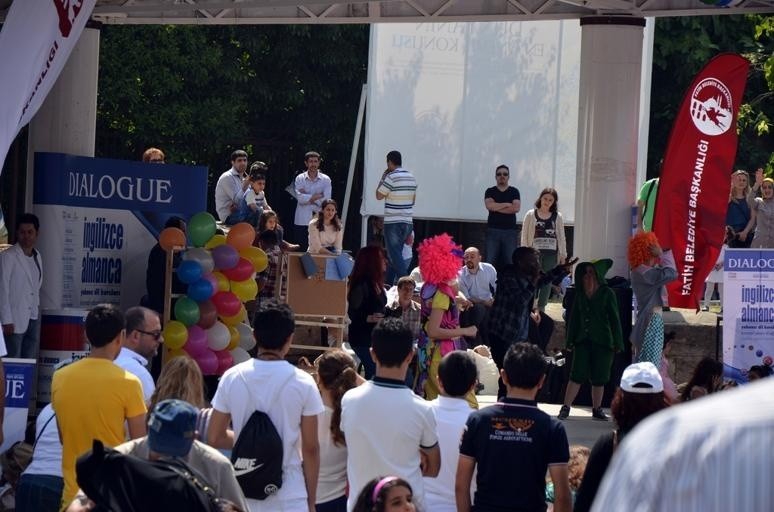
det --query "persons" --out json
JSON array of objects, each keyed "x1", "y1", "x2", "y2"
[
  {"x1": 347, "y1": 216, "x2": 578, "y2": 407},
  {"x1": 376, "y1": 150, "x2": 417, "y2": 287},
  {"x1": 143, "y1": 149, "x2": 164, "y2": 162},
  {"x1": 10, "y1": 302, "x2": 585, "y2": 512},
  {"x1": 637, "y1": 160, "x2": 774, "y2": 314},
  {"x1": 484, "y1": 165, "x2": 520, "y2": 266},
  {"x1": 521, "y1": 188, "x2": 566, "y2": 323},
  {"x1": 293, "y1": 151, "x2": 343, "y2": 257},
  {"x1": 547, "y1": 359, "x2": 774, "y2": 511},
  {"x1": 141, "y1": 217, "x2": 189, "y2": 382},
  {"x1": 214, "y1": 149, "x2": 300, "y2": 294},
  {"x1": 559, "y1": 261, "x2": 622, "y2": 421},
  {"x1": 628, "y1": 232, "x2": 678, "y2": 367},
  {"x1": 0, "y1": 213, "x2": 45, "y2": 358}
]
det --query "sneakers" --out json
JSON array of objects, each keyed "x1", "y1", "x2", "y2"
[
  {"x1": 558, "y1": 405, "x2": 570, "y2": 420},
  {"x1": 282, "y1": 240, "x2": 299, "y2": 251},
  {"x1": 591, "y1": 407, "x2": 608, "y2": 421}
]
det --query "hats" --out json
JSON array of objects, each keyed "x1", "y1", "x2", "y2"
[
  {"x1": 146, "y1": 398, "x2": 196, "y2": 457},
  {"x1": 619, "y1": 361, "x2": 664, "y2": 394}
]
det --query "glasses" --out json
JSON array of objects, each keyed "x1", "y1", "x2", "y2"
[
  {"x1": 135, "y1": 328, "x2": 160, "y2": 340},
  {"x1": 497, "y1": 172, "x2": 508, "y2": 176}
]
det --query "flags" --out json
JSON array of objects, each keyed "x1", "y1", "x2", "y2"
[{"x1": 652, "y1": 54, "x2": 752, "y2": 312}]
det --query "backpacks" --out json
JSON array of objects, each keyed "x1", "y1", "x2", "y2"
[{"x1": 230, "y1": 363, "x2": 296, "y2": 500}]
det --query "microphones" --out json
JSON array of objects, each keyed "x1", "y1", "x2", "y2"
[{"x1": 531, "y1": 265, "x2": 547, "y2": 277}]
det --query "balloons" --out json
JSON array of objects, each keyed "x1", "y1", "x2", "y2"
[{"x1": 160, "y1": 212, "x2": 269, "y2": 374}]
